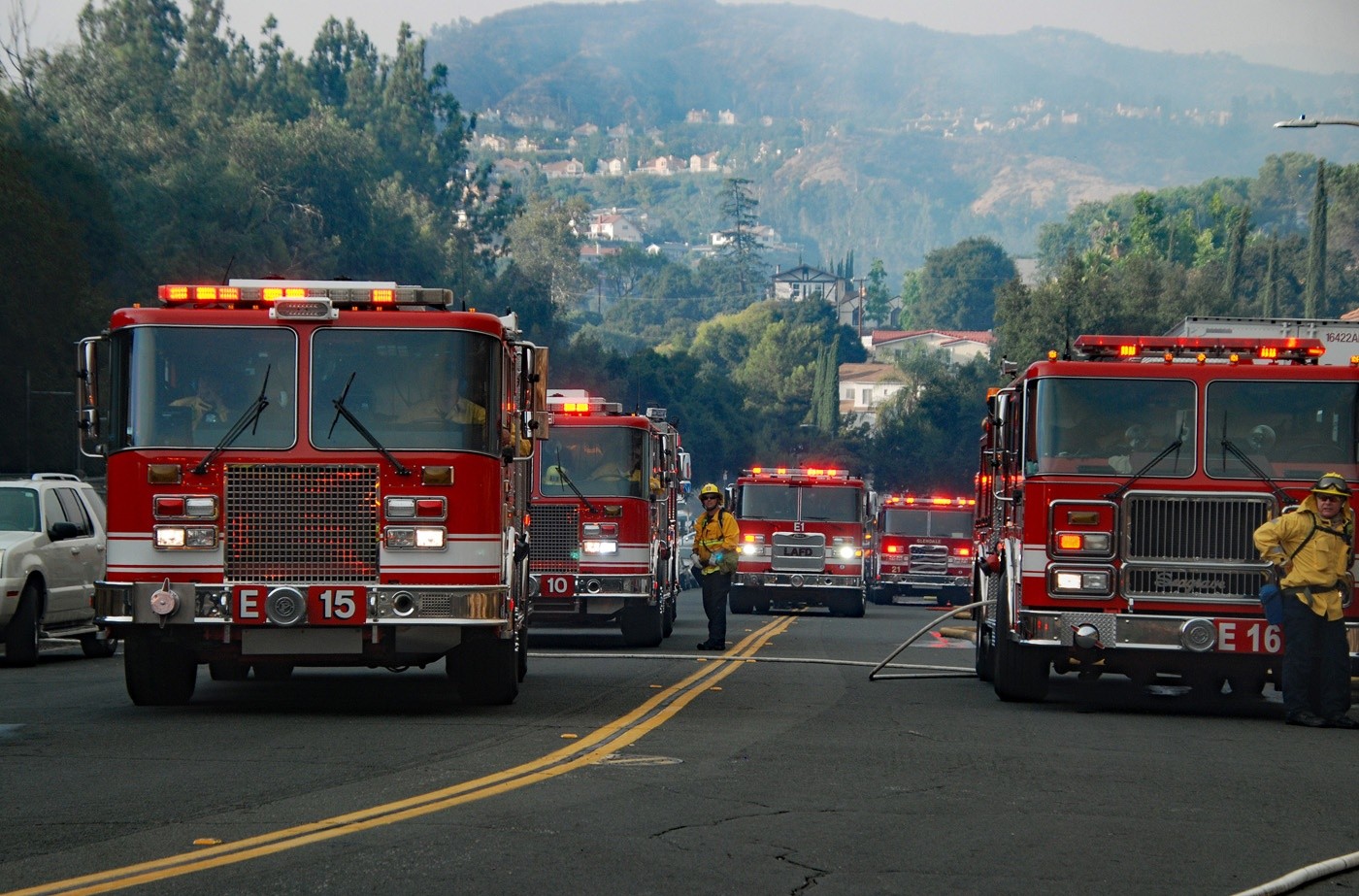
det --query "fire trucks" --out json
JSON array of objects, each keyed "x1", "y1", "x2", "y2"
[
  {"x1": 524, "y1": 390, "x2": 696, "y2": 648},
  {"x1": 69, "y1": 277, "x2": 551, "y2": 708},
  {"x1": 869, "y1": 491, "x2": 979, "y2": 608},
  {"x1": 725, "y1": 465, "x2": 879, "y2": 618},
  {"x1": 960, "y1": 329, "x2": 1359, "y2": 709}
]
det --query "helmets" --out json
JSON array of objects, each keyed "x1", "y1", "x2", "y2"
[
  {"x1": 698, "y1": 483, "x2": 724, "y2": 505},
  {"x1": 1310, "y1": 472, "x2": 1353, "y2": 497}
]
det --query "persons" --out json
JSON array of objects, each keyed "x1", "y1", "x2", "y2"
[
  {"x1": 585, "y1": 447, "x2": 665, "y2": 496},
  {"x1": 680, "y1": 483, "x2": 740, "y2": 651},
  {"x1": 390, "y1": 360, "x2": 531, "y2": 456},
  {"x1": 1253, "y1": 471, "x2": 1359, "y2": 730},
  {"x1": 168, "y1": 366, "x2": 229, "y2": 430}
]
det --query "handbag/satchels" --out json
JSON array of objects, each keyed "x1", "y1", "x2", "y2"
[{"x1": 1259, "y1": 583, "x2": 1283, "y2": 624}]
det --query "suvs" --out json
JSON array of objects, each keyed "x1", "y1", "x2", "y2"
[{"x1": 0, "y1": 473, "x2": 116, "y2": 667}]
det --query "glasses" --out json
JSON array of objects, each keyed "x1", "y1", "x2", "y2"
[
  {"x1": 704, "y1": 496, "x2": 717, "y2": 500},
  {"x1": 1315, "y1": 494, "x2": 1344, "y2": 503},
  {"x1": 1318, "y1": 477, "x2": 1348, "y2": 492}
]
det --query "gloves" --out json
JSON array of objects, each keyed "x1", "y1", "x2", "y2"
[{"x1": 690, "y1": 553, "x2": 703, "y2": 569}]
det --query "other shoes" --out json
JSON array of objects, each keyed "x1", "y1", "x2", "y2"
[
  {"x1": 697, "y1": 640, "x2": 726, "y2": 651},
  {"x1": 1285, "y1": 712, "x2": 1327, "y2": 726},
  {"x1": 1328, "y1": 715, "x2": 1359, "y2": 729}
]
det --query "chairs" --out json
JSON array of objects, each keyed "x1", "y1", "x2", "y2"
[{"x1": 542, "y1": 443, "x2": 571, "y2": 479}]
